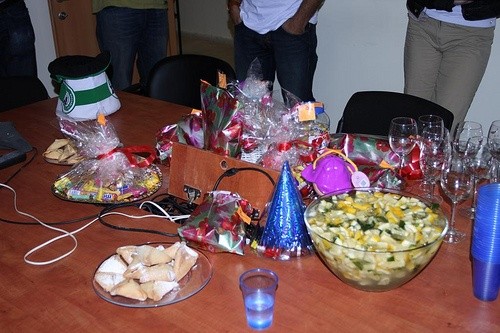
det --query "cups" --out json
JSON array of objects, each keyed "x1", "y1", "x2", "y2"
[
  {"x1": 238, "y1": 268, "x2": 280, "y2": 329},
  {"x1": 471, "y1": 185, "x2": 500, "y2": 302}
]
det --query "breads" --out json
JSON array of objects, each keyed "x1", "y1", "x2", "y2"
[
  {"x1": 42, "y1": 138, "x2": 81, "y2": 164},
  {"x1": 109, "y1": 242, "x2": 199, "y2": 301}
]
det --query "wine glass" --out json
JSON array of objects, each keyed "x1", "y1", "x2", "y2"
[
  {"x1": 417, "y1": 115, "x2": 500, "y2": 245},
  {"x1": 389, "y1": 118, "x2": 418, "y2": 182}
]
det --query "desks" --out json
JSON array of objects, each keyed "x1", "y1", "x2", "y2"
[{"x1": 0, "y1": 88, "x2": 500, "y2": 333}]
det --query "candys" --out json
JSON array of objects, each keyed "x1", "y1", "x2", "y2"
[{"x1": 55, "y1": 169, "x2": 161, "y2": 202}]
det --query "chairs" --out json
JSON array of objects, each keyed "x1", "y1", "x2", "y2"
[
  {"x1": 335, "y1": 91, "x2": 454, "y2": 137},
  {"x1": 140, "y1": 54, "x2": 237, "y2": 111}
]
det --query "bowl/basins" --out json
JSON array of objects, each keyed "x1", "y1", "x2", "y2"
[{"x1": 304, "y1": 188, "x2": 448, "y2": 292}]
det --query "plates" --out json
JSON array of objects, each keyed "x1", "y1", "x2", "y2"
[{"x1": 93, "y1": 243, "x2": 212, "y2": 309}]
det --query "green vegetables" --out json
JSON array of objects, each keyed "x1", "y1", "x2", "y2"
[{"x1": 323, "y1": 199, "x2": 442, "y2": 270}]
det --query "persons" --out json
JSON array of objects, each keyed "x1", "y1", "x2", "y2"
[
  {"x1": 91, "y1": 0, "x2": 170, "y2": 90},
  {"x1": 226, "y1": 0, "x2": 326, "y2": 107},
  {"x1": 403, "y1": 0, "x2": 500, "y2": 137},
  {"x1": 0, "y1": 0, "x2": 51, "y2": 113}
]
